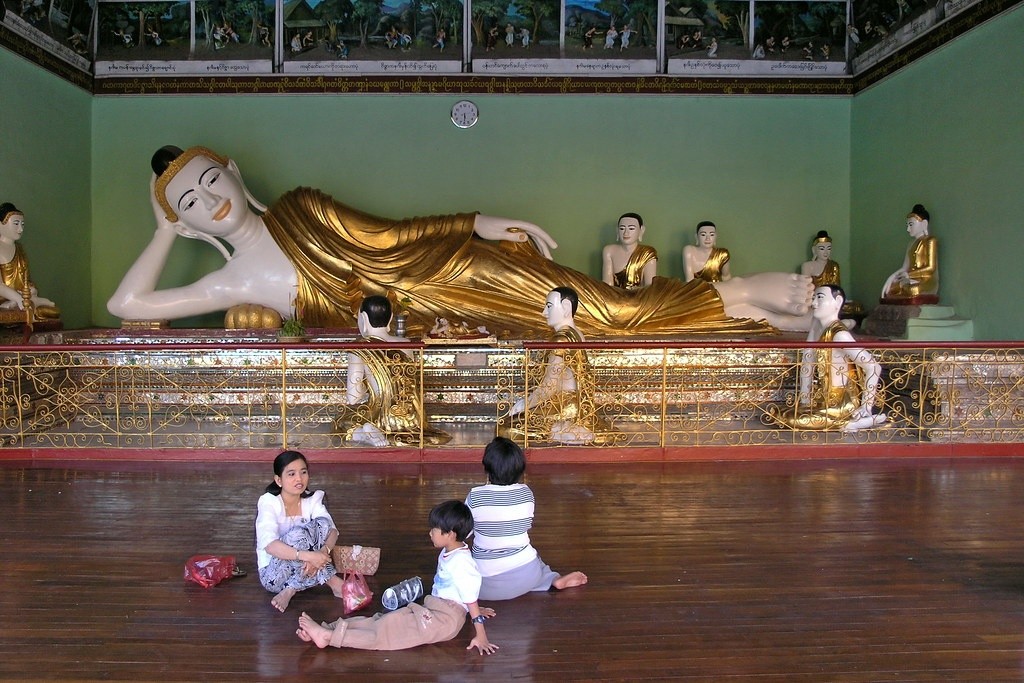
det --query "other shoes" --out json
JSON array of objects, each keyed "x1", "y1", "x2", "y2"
[{"x1": 231, "y1": 566, "x2": 247, "y2": 576}]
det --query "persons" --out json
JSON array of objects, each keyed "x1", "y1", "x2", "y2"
[
  {"x1": 776, "y1": 284, "x2": 886, "y2": 432},
  {"x1": 431, "y1": 315, "x2": 489, "y2": 337},
  {"x1": 0, "y1": 202, "x2": 57, "y2": 311},
  {"x1": 334, "y1": 295, "x2": 452, "y2": 447},
  {"x1": 107, "y1": 144, "x2": 857, "y2": 339},
  {"x1": 297, "y1": 501, "x2": 500, "y2": 655},
  {"x1": 512, "y1": 286, "x2": 626, "y2": 446},
  {"x1": 682, "y1": 220, "x2": 731, "y2": 281},
  {"x1": 464, "y1": 436, "x2": 588, "y2": 601},
  {"x1": 255, "y1": 450, "x2": 373, "y2": 613},
  {"x1": 601, "y1": 213, "x2": 659, "y2": 290},
  {"x1": 880, "y1": 204, "x2": 938, "y2": 305},
  {"x1": 800, "y1": 230, "x2": 853, "y2": 304}
]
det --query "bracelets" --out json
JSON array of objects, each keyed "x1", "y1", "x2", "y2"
[
  {"x1": 324, "y1": 544, "x2": 331, "y2": 555},
  {"x1": 296, "y1": 550, "x2": 299, "y2": 562}
]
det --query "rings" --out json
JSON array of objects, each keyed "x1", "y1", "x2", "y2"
[
  {"x1": 306, "y1": 568, "x2": 310, "y2": 572},
  {"x1": 323, "y1": 563, "x2": 327, "y2": 567}
]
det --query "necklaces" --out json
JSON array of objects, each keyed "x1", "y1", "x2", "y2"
[{"x1": 285, "y1": 496, "x2": 301, "y2": 525}]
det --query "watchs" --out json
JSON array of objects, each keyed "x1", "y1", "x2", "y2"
[{"x1": 470, "y1": 614, "x2": 484, "y2": 625}]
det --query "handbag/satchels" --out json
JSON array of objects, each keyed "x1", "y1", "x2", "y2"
[
  {"x1": 382, "y1": 576, "x2": 424, "y2": 610},
  {"x1": 332, "y1": 545, "x2": 380, "y2": 575},
  {"x1": 341, "y1": 568, "x2": 374, "y2": 613},
  {"x1": 184, "y1": 555, "x2": 236, "y2": 588}
]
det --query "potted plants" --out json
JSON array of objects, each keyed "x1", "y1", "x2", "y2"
[{"x1": 277, "y1": 314, "x2": 306, "y2": 343}]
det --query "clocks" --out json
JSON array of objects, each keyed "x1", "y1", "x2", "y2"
[{"x1": 450, "y1": 99, "x2": 480, "y2": 129}]
